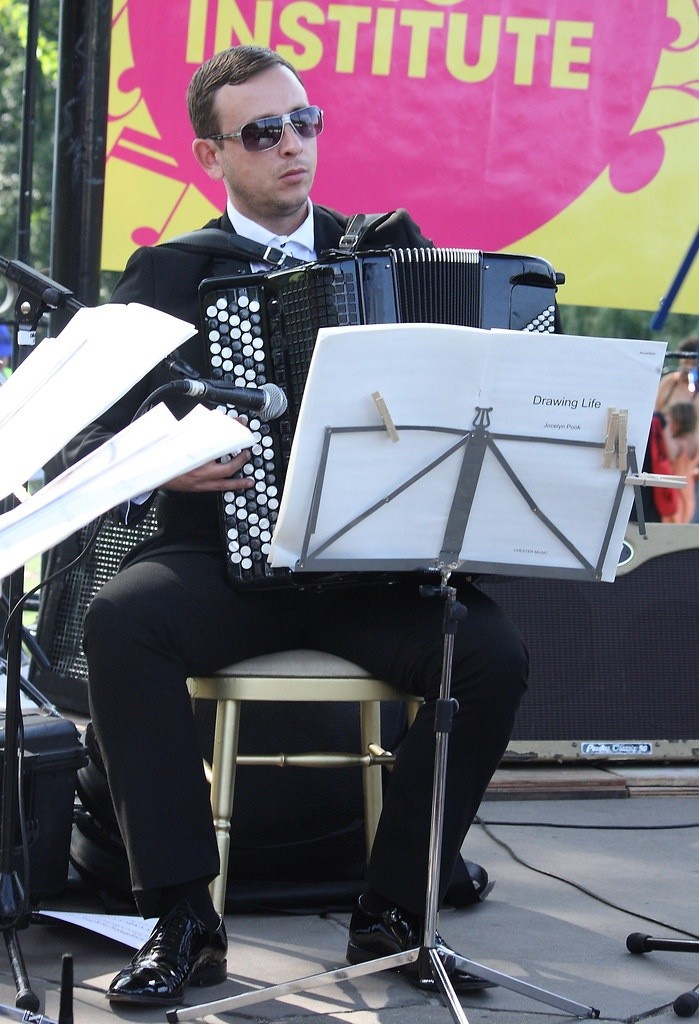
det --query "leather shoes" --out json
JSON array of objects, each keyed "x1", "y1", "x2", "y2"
[
  {"x1": 106, "y1": 901, "x2": 228, "y2": 1005},
  {"x1": 347, "y1": 893, "x2": 500, "y2": 988}
]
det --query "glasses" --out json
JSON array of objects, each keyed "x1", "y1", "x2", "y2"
[{"x1": 214, "y1": 101, "x2": 325, "y2": 152}]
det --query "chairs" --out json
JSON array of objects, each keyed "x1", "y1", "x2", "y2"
[{"x1": 187, "y1": 647, "x2": 426, "y2": 923}]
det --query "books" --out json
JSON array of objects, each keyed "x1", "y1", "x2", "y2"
[{"x1": 268, "y1": 322, "x2": 668, "y2": 585}]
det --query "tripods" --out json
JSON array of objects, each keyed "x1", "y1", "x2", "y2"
[{"x1": 162, "y1": 398, "x2": 640, "y2": 1024}]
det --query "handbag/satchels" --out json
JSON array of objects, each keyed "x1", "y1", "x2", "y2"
[{"x1": 650, "y1": 417, "x2": 678, "y2": 515}]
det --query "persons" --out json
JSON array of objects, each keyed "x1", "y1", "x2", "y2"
[
  {"x1": 652, "y1": 339, "x2": 699, "y2": 521},
  {"x1": 44, "y1": 44, "x2": 534, "y2": 1005}
]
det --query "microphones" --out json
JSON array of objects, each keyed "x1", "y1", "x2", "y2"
[{"x1": 170, "y1": 378, "x2": 288, "y2": 422}]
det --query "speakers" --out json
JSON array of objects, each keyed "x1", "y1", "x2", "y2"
[
  {"x1": 469, "y1": 521, "x2": 698, "y2": 763},
  {"x1": 25, "y1": 487, "x2": 169, "y2": 717}
]
